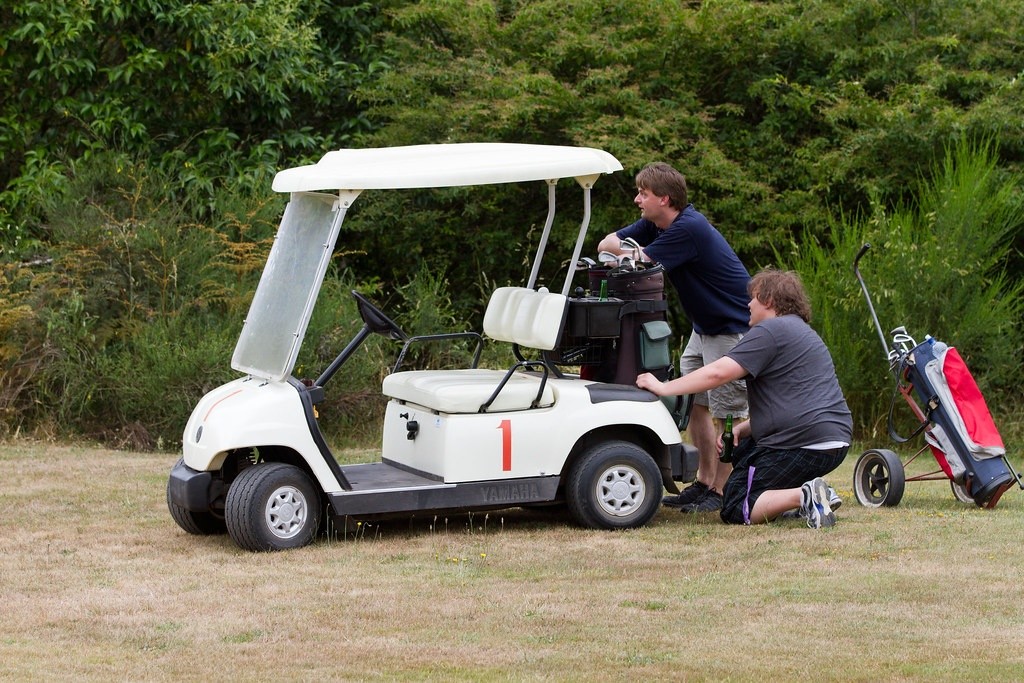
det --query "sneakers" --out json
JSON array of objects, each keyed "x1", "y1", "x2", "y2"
[
  {"x1": 782, "y1": 486, "x2": 842, "y2": 519},
  {"x1": 681, "y1": 487, "x2": 724, "y2": 513},
  {"x1": 662, "y1": 481, "x2": 708, "y2": 507},
  {"x1": 799, "y1": 477, "x2": 835, "y2": 529}
]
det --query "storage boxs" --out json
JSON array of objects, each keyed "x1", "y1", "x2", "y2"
[{"x1": 567, "y1": 297, "x2": 625, "y2": 338}]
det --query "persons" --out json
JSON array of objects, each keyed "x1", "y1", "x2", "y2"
[
  {"x1": 636, "y1": 270, "x2": 854, "y2": 529},
  {"x1": 598, "y1": 162, "x2": 753, "y2": 513}
]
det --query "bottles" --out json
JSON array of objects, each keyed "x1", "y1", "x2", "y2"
[
  {"x1": 925, "y1": 333, "x2": 948, "y2": 361},
  {"x1": 598, "y1": 280, "x2": 610, "y2": 302},
  {"x1": 719, "y1": 414, "x2": 734, "y2": 463}
]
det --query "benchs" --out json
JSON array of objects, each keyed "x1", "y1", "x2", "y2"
[{"x1": 382, "y1": 286, "x2": 569, "y2": 416}]
